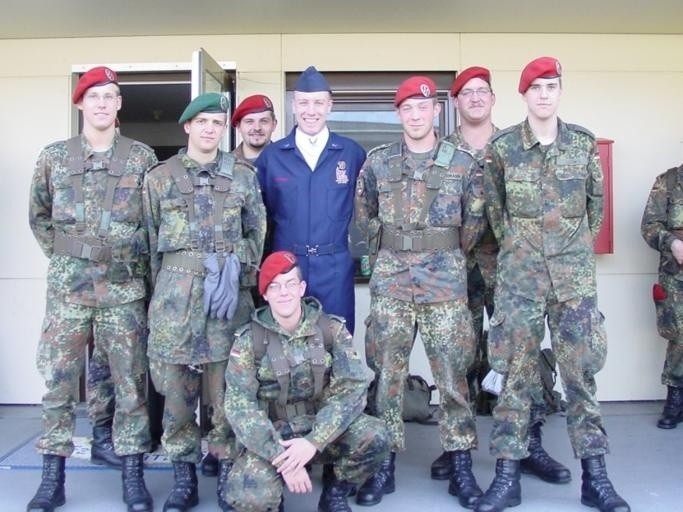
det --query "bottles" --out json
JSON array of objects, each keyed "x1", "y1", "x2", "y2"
[{"x1": 360, "y1": 254, "x2": 371, "y2": 276}]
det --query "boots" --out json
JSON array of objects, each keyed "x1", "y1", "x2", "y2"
[
  {"x1": 26, "y1": 454, "x2": 67, "y2": 512},
  {"x1": 162, "y1": 460, "x2": 199, "y2": 512},
  {"x1": 473, "y1": 458, "x2": 522, "y2": 512},
  {"x1": 520, "y1": 422, "x2": 573, "y2": 484},
  {"x1": 356, "y1": 451, "x2": 398, "y2": 506},
  {"x1": 657, "y1": 386, "x2": 683, "y2": 429},
  {"x1": 90, "y1": 425, "x2": 122, "y2": 469},
  {"x1": 580, "y1": 453, "x2": 631, "y2": 512},
  {"x1": 448, "y1": 450, "x2": 484, "y2": 509},
  {"x1": 216, "y1": 458, "x2": 236, "y2": 512},
  {"x1": 120, "y1": 453, "x2": 155, "y2": 512},
  {"x1": 430, "y1": 451, "x2": 451, "y2": 480},
  {"x1": 317, "y1": 471, "x2": 353, "y2": 512},
  {"x1": 201, "y1": 452, "x2": 219, "y2": 477}
]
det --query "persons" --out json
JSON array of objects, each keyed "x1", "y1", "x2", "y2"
[
  {"x1": 26, "y1": 67, "x2": 158, "y2": 512},
  {"x1": 142, "y1": 91, "x2": 266, "y2": 511},
  {"x1": 229, "y1": 94, "x2": 278, "y2": 165},
  {"x1": 349, "y1": 75, "x2": 482, "y2": 509},
  {"x1": 251, "y1": 66, "x2": 367, "y2": 344},
  {"x1": 430, "y1": 67, "x2": 572, "y2": 486},
  {"x1": 475, "y1": 56, "x2": 631, "y2": 512},
  {"x1": 87, "y1": 339, "x2": 124, "y2": 469},
  {"x1": 222, "y1": 250, "x2": 390, "y2": 511},
  {"x1": 639, "y1": 163, "x2": 683, "y2": 429}
]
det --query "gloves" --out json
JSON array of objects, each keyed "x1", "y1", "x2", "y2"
[
  {"x1": 211, "y1": 252, "x2": 242, "y2": 322},
  {"x1": 202, "y1": 253, "x2": 220, "y2": 316}
]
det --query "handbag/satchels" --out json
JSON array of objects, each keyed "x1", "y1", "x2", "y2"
[{"x1": 366, "y1": 372, "x2": 438, "y2": 426}]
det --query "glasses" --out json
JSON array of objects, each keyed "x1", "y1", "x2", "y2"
[{"x1": 458, "y1": 88, "x2": 488, "y2": 97}]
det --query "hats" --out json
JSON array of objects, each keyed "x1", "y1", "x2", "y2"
[
  {"x1": 294, "y1": 65, "x2": 332, "y2": 93},
  {"x1": 231, "y1": 94, "x2": 275, "y2": 128},
  {"x1": 519, "y1": 56, "x2": 562, "y2": 93},
  {"x1": 258, "y1": 250, "x2": 298, "y2": 296},
  {"x1": 394, "y1": 75, "x2": 437, "y2": 107},
  {"x1": 72, "y1": 66, "x2": 119, "y2": 104},
  {"x1": 178, "y1": 92, "x2": 230, "y2": 125},
  {"x1": 450, "y1": 66, "x2": 490, "y2": 97}
]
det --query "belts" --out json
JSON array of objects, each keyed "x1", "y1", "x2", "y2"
[
  {"x1": 271, "y1": 241, "x2": 346, "y2": 257},
  {"x1": 52, "y1": 229, "x2": 110, "y2": 263},
  {"x1": 380, "y1": 230, "x2": 461, "y2": 253},
  {"x1": 160, "y1": 250, "x2": 225, "y2": 278},
  {"x1": 268, "y1": 399, "x2": 316, "y2": 422}
]
